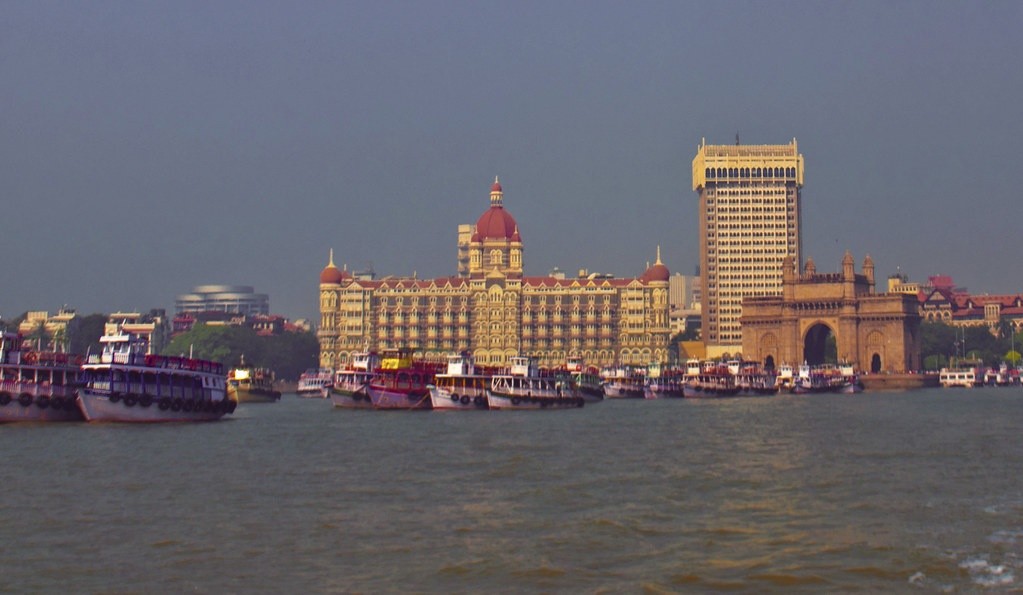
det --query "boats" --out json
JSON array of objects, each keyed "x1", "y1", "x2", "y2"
[
  {"x1": 0, "y1": 330, "x2": 85, "y2": 422},
  {"x1": 71, "y1": 318, "x2": 234, "y2": 424},
  {"x1": 295, "y1": 346, "x2": 865, "y2": 411},
  {"x1": 938, "y1": 361, "x2": 1023, "y2": 389},
  {"x1": 225, "y1": 365, "x2": 282, "y2": 405}
]
